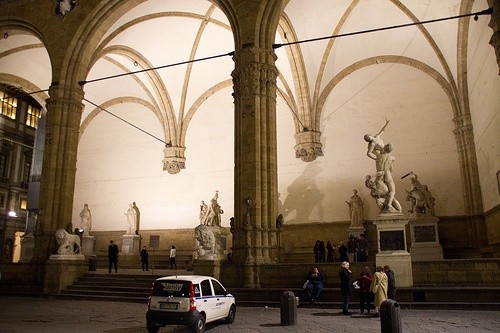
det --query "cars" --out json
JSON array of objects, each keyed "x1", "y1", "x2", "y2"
[{"x1": 146, "y1": 275, "x2": 236, "y2": 333}]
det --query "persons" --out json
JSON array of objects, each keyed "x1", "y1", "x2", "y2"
[
  {"x1": 79, "y1": 204, "x2": 92, "y2": 236},
  {"x1": 140, "y1": 246, "x2": 150, "y2": 272},
  {"x1": 364, "y1": 117, "x2": 401, "y2": 214},
  {"x1": 357, "y1": 263, "x2": 396, "y2": 316},
  {"x1": 338, "y1": 261, "x2": 354, "y2": 316},
  {"x1": 304, "y1": 267, "x2": 324, "y2": 303},
  {"x1": 404, "y1": 171, "x2": 436, "y2": 217},
  {"x1": 65, "y1": 223, "x2": 82, "y2": 254},
  {"x1": 345, "y1": 190, "x2": 364, "y2": 227},
  {"x1": 169, "y1": 246, "x2": 178, "y2": 270},
  {"x1": 196, "y1": 194, "x2": 223, "y2": 227},
  {"x1": 313, "y1": 234, "x2": 369, "y2": 263},
  {"x1": 123, "y1": 204, "x2": 138, "y2": 236},
  {"x1": 108, "y1": 240, "x2": 119, "y2": 274}
]
